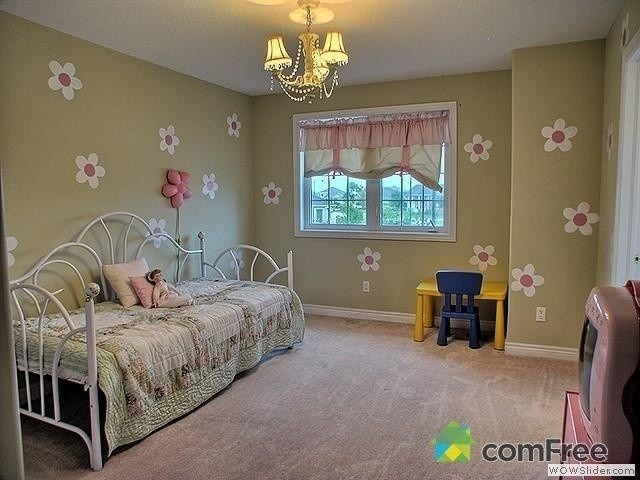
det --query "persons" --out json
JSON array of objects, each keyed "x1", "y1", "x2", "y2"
[{"x1": 146, "y1": 269, "x2": 194, "y2": 308}]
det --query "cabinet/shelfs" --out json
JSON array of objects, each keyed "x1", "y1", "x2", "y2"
[{"x1": 559, "y1": 389, "x2": 611, "y2": 480}]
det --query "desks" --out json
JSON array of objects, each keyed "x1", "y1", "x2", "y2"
[{"x1": 414, "y1": 282, "x2": 508, "y2": 351}]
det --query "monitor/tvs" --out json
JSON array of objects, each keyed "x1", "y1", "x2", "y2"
[{"x1": 577, "y1": 280, "x2": 640, "y2": 464}]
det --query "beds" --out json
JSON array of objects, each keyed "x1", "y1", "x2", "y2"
[{"x1": 10, "y1": 211, "x2": 305, "y2": 471}]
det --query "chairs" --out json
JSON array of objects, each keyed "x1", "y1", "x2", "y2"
[{"x1": 437, "y1": 269, "x2": 483, "y2": 348}]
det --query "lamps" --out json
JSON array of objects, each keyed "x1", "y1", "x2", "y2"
[{"x1": 264, "y1": 0, "x2": 348, "y2": 105}]
